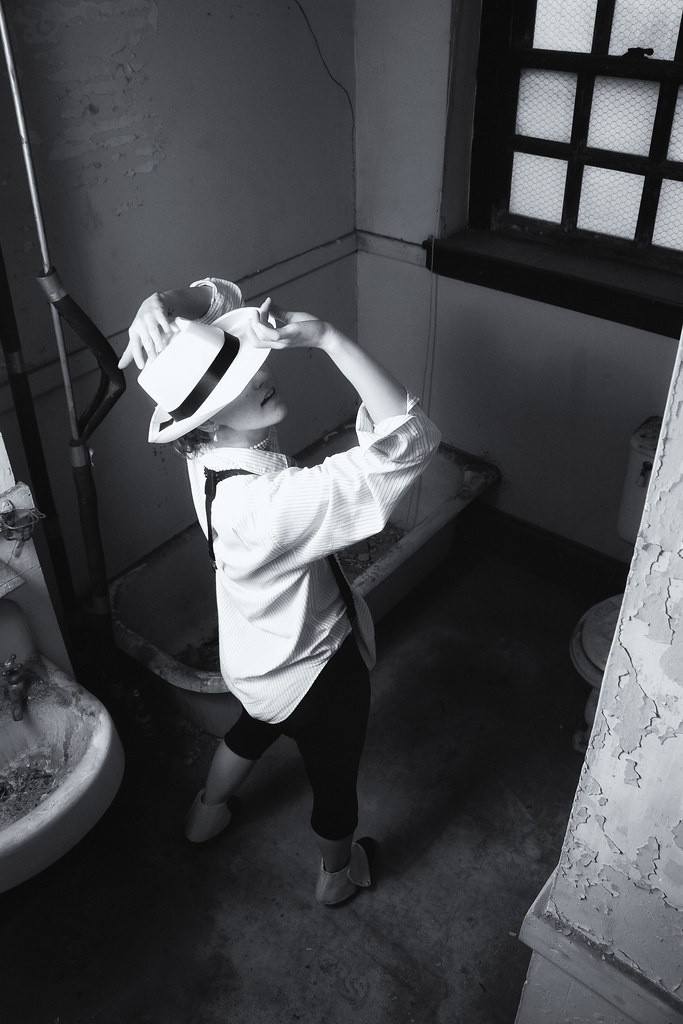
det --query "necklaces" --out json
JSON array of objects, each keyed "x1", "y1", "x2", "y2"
[{"x1": 247, "y1": 426, "x2": 276, "y2": 454}]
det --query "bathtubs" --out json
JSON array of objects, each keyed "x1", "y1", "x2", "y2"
[{"x1": 106, "y1": 415, "x2": 504, "y2": 742}]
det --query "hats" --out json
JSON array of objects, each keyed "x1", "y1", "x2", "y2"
[{"x1": 138, "y1": 308, "x2": 277, "y2": 444}]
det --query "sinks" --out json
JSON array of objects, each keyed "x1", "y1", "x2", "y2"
[{"x1": 1, "y1": 649, "x2": 127, "y2": 892}]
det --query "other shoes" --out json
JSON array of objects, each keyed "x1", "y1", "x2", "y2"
[
  {"x1": 178, "y1": 791, "x2": 241, "y2": 851},
  {"x1": 314, "y1": 835, "x2": 378, "y2": 907}
]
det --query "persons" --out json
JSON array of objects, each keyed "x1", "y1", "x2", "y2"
[{"x1": 111, "y1": 279, "x2": 444, "y2": 907}]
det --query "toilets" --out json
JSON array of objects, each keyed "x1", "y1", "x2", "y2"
[{"x1": 569, "y1": 414, "x2": 662, "y2": 744}]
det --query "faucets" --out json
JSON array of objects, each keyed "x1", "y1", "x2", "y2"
[{"x1": 4, "y1": 653, "x2": 31, "y2": 725}]
